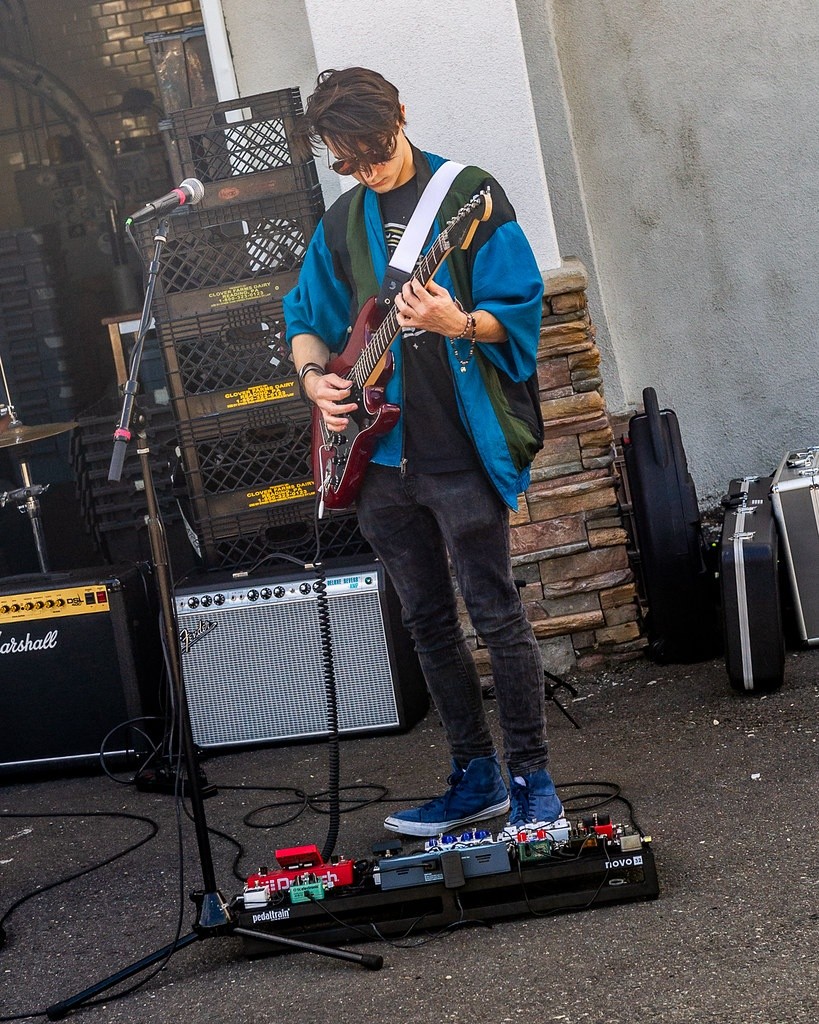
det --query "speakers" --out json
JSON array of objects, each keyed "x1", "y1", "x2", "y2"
[
  {"x1": 171, "y1": 553, "x2": 428, "y2": 757},
  {"x1": 0, "y1": 560, "x2": 159, "y2": 785}
]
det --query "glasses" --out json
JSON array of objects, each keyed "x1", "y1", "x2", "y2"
[{"x1": 326, "y1": 134, "x2": 392, "y2": 176}]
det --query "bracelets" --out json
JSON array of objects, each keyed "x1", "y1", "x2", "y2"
[
  {"x1": 450, "y1": 310, "x2": 476, "y2": 374},
  {"x1": 298, "y1": 362, "x2": 325, "y2": 399}
]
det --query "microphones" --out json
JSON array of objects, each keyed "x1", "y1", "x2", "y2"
[{"x1": 126, "y1": 178, "x2": 204, "y2": 224}]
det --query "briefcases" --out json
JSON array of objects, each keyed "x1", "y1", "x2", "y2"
[{"x1": 721, "y1": 446, "x2": 819, "y2": 691}]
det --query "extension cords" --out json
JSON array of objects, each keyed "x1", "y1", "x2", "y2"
[{"x1": 136, "y1": 772, "x2": 218, "y2": 800}]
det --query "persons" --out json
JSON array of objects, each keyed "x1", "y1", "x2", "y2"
[{"x1": 283, "y1": 67, "x2": 565, "y2": 842}]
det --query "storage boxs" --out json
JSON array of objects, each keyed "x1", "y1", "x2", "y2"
[{"x1": 0, "y1": 88, "x2": 375, "y2": 568}]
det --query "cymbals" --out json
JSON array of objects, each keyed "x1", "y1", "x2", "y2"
[{"x1": 0, "y1": 421, "x2": 82, "y2": 451}]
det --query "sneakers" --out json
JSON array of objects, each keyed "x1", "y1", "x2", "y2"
[
  {"x1": 384, "y1": 749, "x2": 510, "y2": 837},
  {"x1": 507, "y1": 769, "x2": 565, "y2": 830}
]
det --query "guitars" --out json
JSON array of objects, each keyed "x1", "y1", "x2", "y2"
[{"x1": 310, "y1": 184, "x2": 494, "y2": 519}]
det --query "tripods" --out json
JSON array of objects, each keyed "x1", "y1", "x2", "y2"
[{"x1": 41, "y1": 216, "x2": 385, "y2": 1024}]
